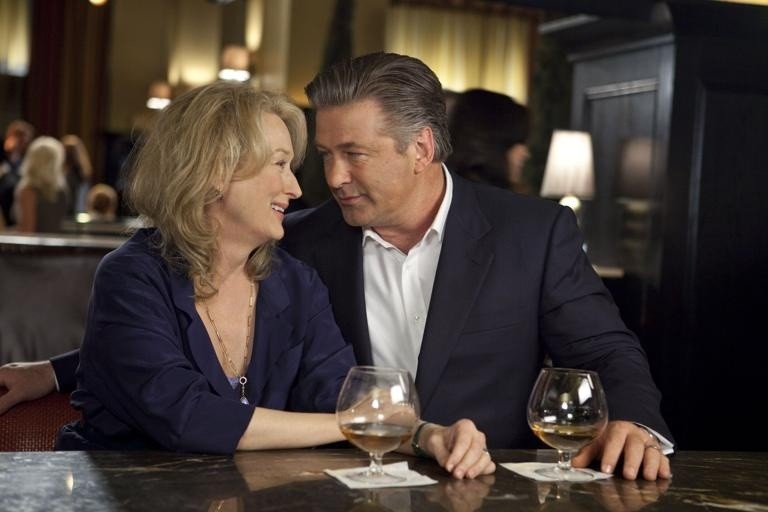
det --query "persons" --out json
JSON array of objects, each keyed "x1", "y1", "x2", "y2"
[
  {"x1": 0, "y1": 50, "x2": 680, "y2": 486},
  {"x1": 52, "y1": 79, "x2": 498, "y2": 484},
  {"x1": 0, "y1": 121, "x2": 129, "y2": 237}
]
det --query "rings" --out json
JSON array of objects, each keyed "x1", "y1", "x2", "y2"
[
  {"x1": 483, "y1": 447, "x2": 492, "y2": 459},
  {"x1": 646, "y1": 444, "x2": 662, "y2": 454}
]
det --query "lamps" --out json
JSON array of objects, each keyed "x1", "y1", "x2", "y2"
[
  {"x1": 541, "y1": 127, "x2": 598, "y2": 213},
  {"x1": 144, "y1": 78, "x2": 173, "y2": 110},
  {"x1": 217, "y1": 42, "x2": 253, "y2": 82},
  {"x1": 613, "y1": 132, "x2": 655, "y2": 265}
]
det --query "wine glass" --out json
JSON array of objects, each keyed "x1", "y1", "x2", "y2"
[
  {"x1": 525, "y1": 366, "x2": 608, "y2": 482},
  {"x1": 335, "y1": 365, "x2": 421, "y2": 484}
]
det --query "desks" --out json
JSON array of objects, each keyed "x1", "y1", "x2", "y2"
[{"x1": 0, "y1": 444, "x2": 765, "y2": 511}]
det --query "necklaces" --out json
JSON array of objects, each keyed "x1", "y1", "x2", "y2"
[{"x1": 202, "y1": 282, "x2": 254, "y2": 407}]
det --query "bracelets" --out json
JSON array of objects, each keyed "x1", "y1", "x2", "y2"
[{"x1": 412, "y1": 421, "x2": 431, "y2": 459}]
